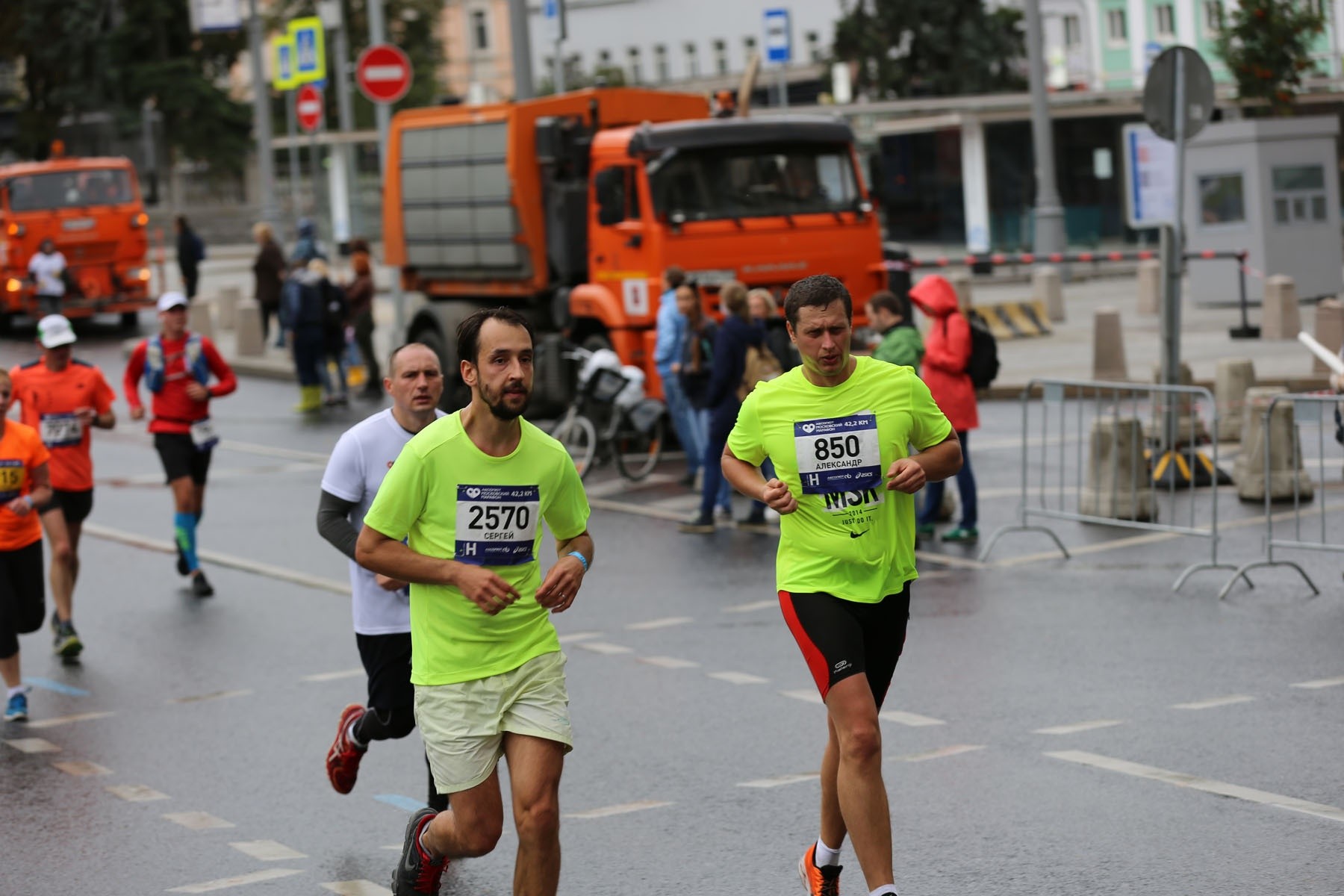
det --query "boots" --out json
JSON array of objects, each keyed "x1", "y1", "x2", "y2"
[{"x1": 299, "y1": 386, "x2": 322, "y2": 412}]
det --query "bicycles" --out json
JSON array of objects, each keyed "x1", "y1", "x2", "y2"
[{"x1": 546, "y1": 337, "x2": 667, "y2": 484}]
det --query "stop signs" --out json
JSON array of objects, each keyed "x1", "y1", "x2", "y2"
[
  {"x1": 295, "y1": 86, "x2": 322, "y2": 130},
  {"x1": 354, "y1": 42, "x2": 415, "y2": 104}
]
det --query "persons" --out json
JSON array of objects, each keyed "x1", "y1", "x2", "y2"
[
  {"x1": 316, "y1": 341, "x2": 452, "y2": 813},
  {"x1": 174, "y1": 217, "x2": 200, "y2": 299},
  {"x1": 27, "y1": 238, "x2": 68, "y2": 318},
  {"x1": 10, "y1": 314, "x2": 118, "y2": 658},
  {"x1": 0, "y1": 367, "x2": 54, "y2": 723},
  {"x1": 864, "y1": 275, "x2": 980, "y2": 545},
  {"x1": 123, "y1": 290, "x2": 238, "y2": 597},
  {"x1": 654, "y1": 268, "x2": 793, "y2": 535},
  {"x1": 721, "y1": 274, "x2": 965, "y2": 895},
  {"x1": 354, "y1": 307, "x2": 596, "y2": 896},
  {"x1": 252, "y1": 216, "x2": 384, "y2": 415}
]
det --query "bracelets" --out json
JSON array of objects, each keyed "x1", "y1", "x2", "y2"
[
  {"x1": 23, "y1": 494, "x2": 35, "y2": 509},
  {"x1": 568, "y1": 550, "x2": 588, "y2": 573}
]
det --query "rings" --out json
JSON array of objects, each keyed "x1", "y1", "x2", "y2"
[{"x1": 559, "y1": 592, "x2": 567, "y2": 600}]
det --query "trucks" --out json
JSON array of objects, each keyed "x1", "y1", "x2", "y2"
[
  {"x1": 0, "y1": 139, "x2": 158, "y2": 338},
  {"x1": 383, "y1": 84, "x2": 890, "y2": 426}
]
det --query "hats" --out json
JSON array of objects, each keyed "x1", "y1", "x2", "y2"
[
  {"x1": 36, "y1": 314, "x2": 77, "y2": 348},
  {"x1": 158, "y1": 292, "x2": 189, "y2": 314}
]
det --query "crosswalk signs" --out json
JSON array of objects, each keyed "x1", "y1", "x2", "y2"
[
  {"x1": 269, "y1": 35, "x2": 300, "y2": 91},
  {"x1": 286, "y1": 15, "x2": 326, "y2": 85}
]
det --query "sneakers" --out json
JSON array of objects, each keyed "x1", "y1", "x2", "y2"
[
  {"x1": 798, "y1": 841, "x2": 844, "y2": 896},
  {"x1": 327, "y1": 707, "x2": 370, "y2": 794},
  {"x1": 391, "y1": 807, "x2": 451, "y2": 896}
]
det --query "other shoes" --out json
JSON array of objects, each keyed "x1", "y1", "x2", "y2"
[
  {"x1": 739, "y1": 512, "x2": 769, "y2": 528},
  {"x1": 942, "y1": 526, "x2": 977, "y2": 544},
  {"x1": 916, "y1": 527, "x2": 935, "y2": 540},
  {"x1": 177, "y1": 540, "x2": 191, "y2": 576},
  {"x1": 192, "y1": 571, "x2": 213, "y2": 594},
  {"x1": 4, "y1": 693, "x2": 27, "y2": 722},
  {"x1": 53, "y1": 626, "x2": 84, "y2": 657},
  {"x1": 679, "y1": 514, "x2": 717, "y2": 533},
  {"x1": 50, "y1": 612, "x2": 61, "y2": 633}
]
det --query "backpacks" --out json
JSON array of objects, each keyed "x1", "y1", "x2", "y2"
[
  {"x1": 944, "y1": 308, "x2": 999, "y2": 388},
  {"x1": 722, "y1": 319, "x2": 783, "y2": 405}
]
